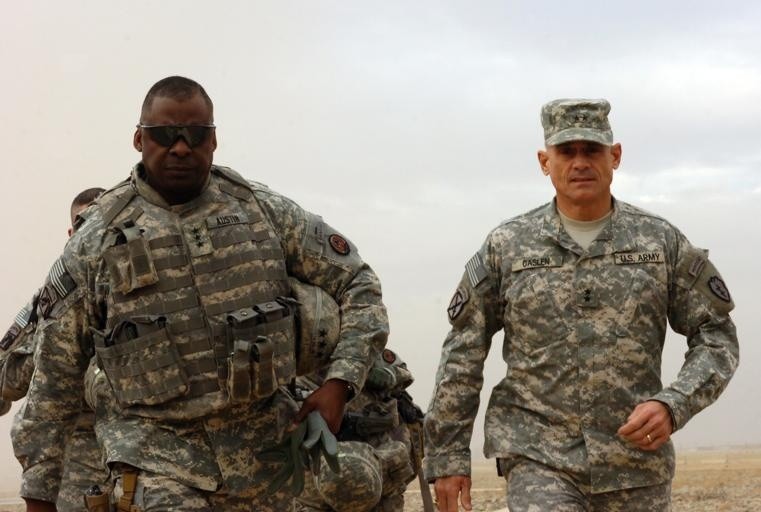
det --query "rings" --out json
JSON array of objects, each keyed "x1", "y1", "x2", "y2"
[{"x1": 645, "y1": 434, "x2": 653, "y2": 443}]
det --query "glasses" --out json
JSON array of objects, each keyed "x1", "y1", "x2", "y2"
[{"x1": 138, "y1": 124, "x2": 216, "y2": 148}]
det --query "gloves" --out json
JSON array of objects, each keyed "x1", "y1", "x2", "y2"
[{"x1": 266, "y1": 409, "x2": 343, "y2": 498}]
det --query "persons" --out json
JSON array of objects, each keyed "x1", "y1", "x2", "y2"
[
  {"x1": 0, "y1": 187, "x2": 110, "y2": 512},
  {"x1": 9, "y1": 76, "x2": 387, "y2": 512},
  {"x1": 422, "y1": 97, "x2": 739, "y2": 512}
]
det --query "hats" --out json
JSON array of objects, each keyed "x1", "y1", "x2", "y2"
[{"x1": 540, "y1": 98, "x2": 614, "y2": 147}]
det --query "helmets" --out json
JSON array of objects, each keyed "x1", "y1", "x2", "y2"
[
  {"x1": 312, "y1": 438, "x2": 383, "y2": 512},
  {"x1": 290, "y1": 276, "x2": 340, "y2": 377}
]
